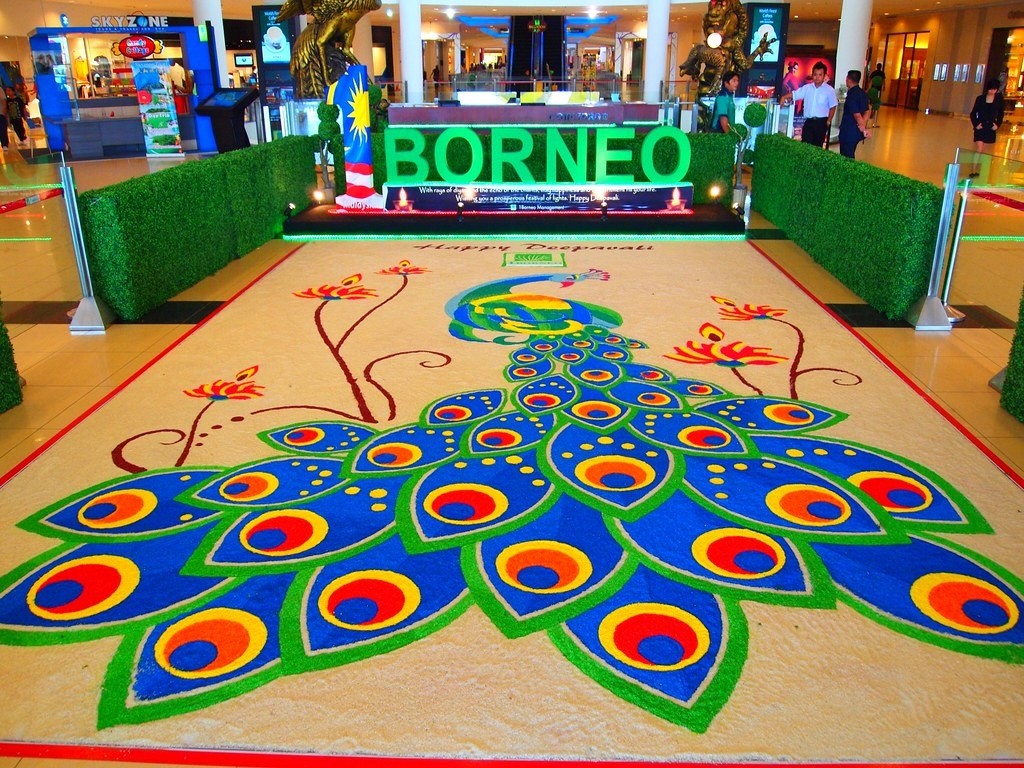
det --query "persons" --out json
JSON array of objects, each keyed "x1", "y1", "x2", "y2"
[
  {"x1": 251, "y1": 66, "x2": 257, "y2": 82},
  {"x1": 430, "y1": 65, "x2": 439, "y2": 95},
  {"x1": 0, "y1": 75, "x2": 37, "y2": 150},
  {"x1": 868, "y1": 63, "x2": 886, "y2": 118},
  {"x1": 780, "y1": 62, "x2": 838, "y2": 149},
  {"x1": 969, "y1": 79, "x2": 1004, "y2": 178},
  {"x1": 461, "y1": 62, "x2": 505, "y2": 73},
  {"x1": 783, "y1": 61, "x2": 802, "y2": 115},
  {"x1": 36, "y1": 54, "x2": 55, "y2": 74},
  {"x1": 839, "y1": 70, "x2": 871, "y2": 158},
  {"x1": 711, "y1": 71, "x2": 739, "y2": 134}
]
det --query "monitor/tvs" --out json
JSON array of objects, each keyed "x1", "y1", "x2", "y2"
[
  {"x1": 234, "y1": 53, "x2": 253, "y2": 66},
  {"x1": 202, "y1": 90, "x2": 248, "y2": 106},
  {"x1": 438, "y1": 101, "x2": 460, "y2": 107}
]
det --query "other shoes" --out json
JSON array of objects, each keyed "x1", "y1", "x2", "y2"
[
  {"x1": 969, "y1": 172, "x2": 979, "y2": 178},
  {"x1": 873, "y1": 123, "x2": 880, "y2": 127}
]
